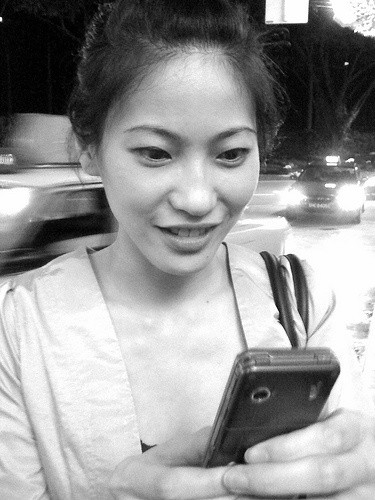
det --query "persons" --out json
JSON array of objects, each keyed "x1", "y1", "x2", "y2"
[{"x1": 0, "y1": 0, "x2": 375, "y2": 500}]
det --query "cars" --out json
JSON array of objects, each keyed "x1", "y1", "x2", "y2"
[{"x1": 285, "y1": 155, "x2": 368, "y2": 225}]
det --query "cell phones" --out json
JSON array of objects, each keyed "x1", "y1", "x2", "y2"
[{"x1": 200, "y1": 346, "x2": 340, "y2": 467}]
